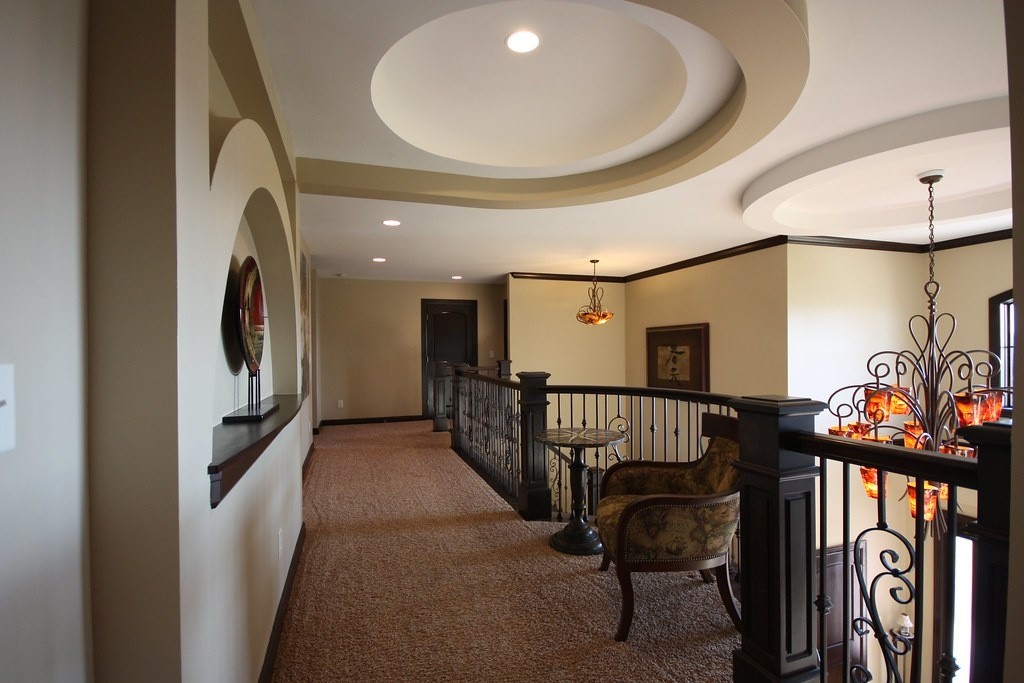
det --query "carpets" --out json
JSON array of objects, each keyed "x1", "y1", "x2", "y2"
[{"x1": 270, "y1": 418, "x2": 743, "y2": 682}]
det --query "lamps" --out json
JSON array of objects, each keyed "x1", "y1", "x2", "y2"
[
  {"x1": 576, "y1": 259, "x2": 614, "y2": 326},
  {"x1": 828, "y1": 169, "x2": 1005, "y2": 542},
  {"x1": 897, "y1": 613, "x2": 913, "y2": 637}
]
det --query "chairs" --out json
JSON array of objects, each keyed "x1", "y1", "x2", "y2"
[{"x1": 594, "y1": 413, "x2": 748, "y2": 642}]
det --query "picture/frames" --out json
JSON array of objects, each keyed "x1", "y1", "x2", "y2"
[{"x1": 646, "y1": 323, "x2": 711, "y2": 405}]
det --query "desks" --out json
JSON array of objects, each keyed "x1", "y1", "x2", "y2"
[{"x1": 534, "y1": 427, "x2": 625, "y2": 556}]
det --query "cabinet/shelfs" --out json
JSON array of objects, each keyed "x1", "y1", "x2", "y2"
[{"x1": 889, "y1": 628, "x2": 915, "y2": 683}]
